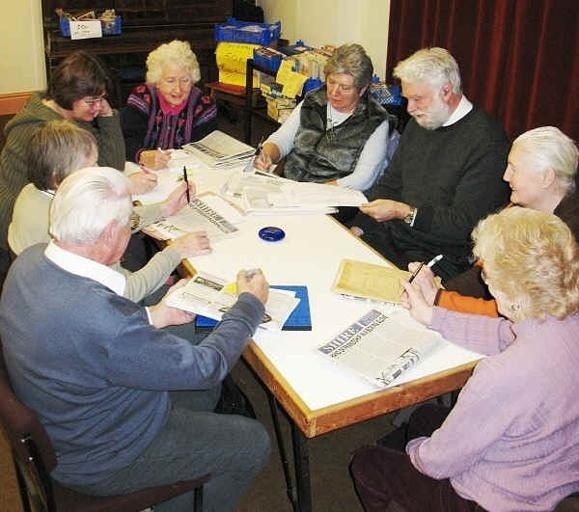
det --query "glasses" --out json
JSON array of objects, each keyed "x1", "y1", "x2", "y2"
[
  {"x1": 480, "y1": 270, "x2": 492, "y2": 285},
  {"x1": 83, "y1": 97, "x2": 103, "y2": 104}
]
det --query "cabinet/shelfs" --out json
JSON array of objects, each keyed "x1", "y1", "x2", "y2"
[{"x1": 42, "y1": 0, "x2": 264, "y2": 108}]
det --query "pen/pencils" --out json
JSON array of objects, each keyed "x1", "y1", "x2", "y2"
[
  {"x1": 400, "y1": 254, "x2": 444, "y2": 296},
  {"x1": 258, "y1": 143, "x2": 269, "y2": 174},
  {"x1": 139, "y1": 165, "x2": 152, "y2": 174},
  {"x1": 183, "y1": 166, "x2": 189, "y2": 203},
  {"x1": 245, "y1": 268, "x2": 262, "y2": 277},
  {"x1": 157, "y1": 147, "x2": 162, "y2": 151}
]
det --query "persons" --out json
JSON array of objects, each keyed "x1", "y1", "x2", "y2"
[{"x1": 1, "y1": 39, "x2": 579, "y2": 511}]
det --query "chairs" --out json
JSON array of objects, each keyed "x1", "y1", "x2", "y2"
[
  {"x1": 0, "y1": 371, "x2": 256, "y2": 512},
  {"x1": 205, "y1": 37, "x2": 289, "y2": 145}
]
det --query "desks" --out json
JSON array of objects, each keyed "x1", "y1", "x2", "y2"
[
  {"x1": 245, "y1": 58, "x2": 409, "y2": 145},
  {"x1": 124, "y1": 140, "x2": 487, "y2": 510}
]
